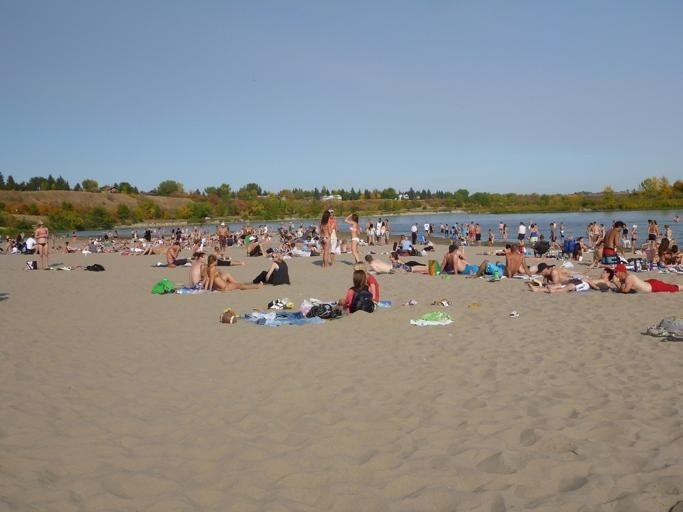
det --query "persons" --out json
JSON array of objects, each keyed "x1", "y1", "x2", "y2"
[{"x1": 1, "y1": 208, "x2": 682, "y2": 314}]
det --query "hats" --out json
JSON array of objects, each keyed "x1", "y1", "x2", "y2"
[
  {"x1": 536, "y1": 263, "x2": 547, "y2": 274},
  {"x1": 613, "y1": 264, "x2": 627, "y2": 273}
]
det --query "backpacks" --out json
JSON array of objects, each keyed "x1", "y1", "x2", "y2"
[{"x1": 350, "y1": 287, "x2": 374, "y2": 313}]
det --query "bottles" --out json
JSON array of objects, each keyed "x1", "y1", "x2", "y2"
[{"x1": 494, "y1": 269, "x2": 499, "y2": 280}]
